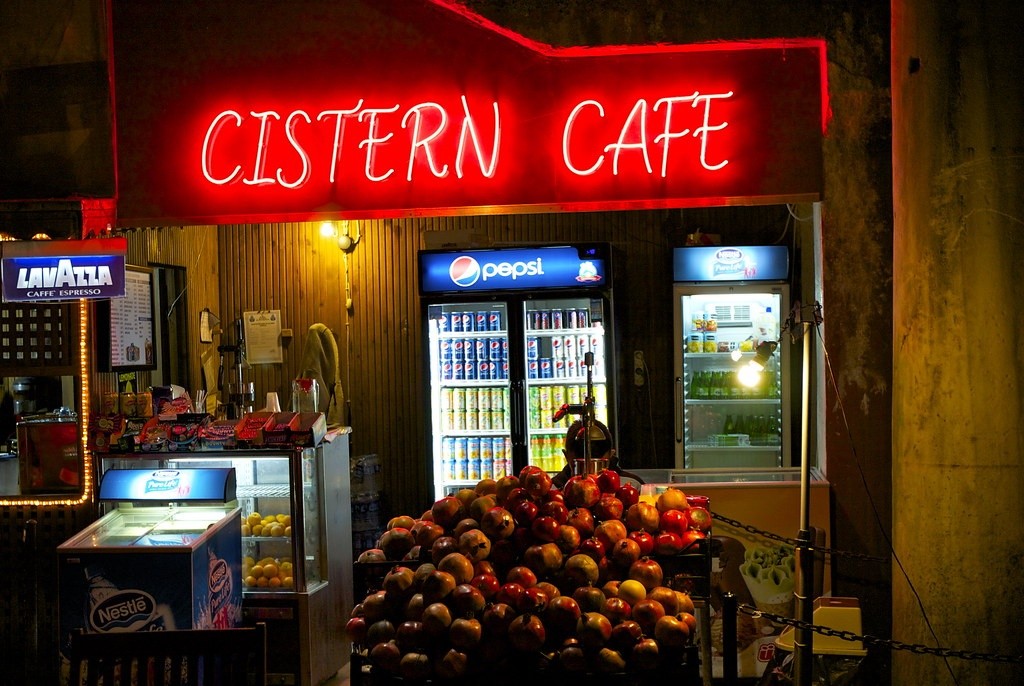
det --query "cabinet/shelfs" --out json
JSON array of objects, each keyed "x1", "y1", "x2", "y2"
[{"x1": 94, "y1": 427, "x2": 359, "y2": 686}]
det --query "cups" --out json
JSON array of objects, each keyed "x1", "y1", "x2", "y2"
[
  {"x1": 574, "y1": 459, "x2": 608, "y2": 476},
  {"x1": 265, "y1": 392, "x2": 281, "y2": 412},
  {"x1": 59, "y1": 406, "x2": 72, "y2": 422},
  {"x1": 52, "y1": 409, "x2": 59, "y2": 420},
  {"x1": 189, "y1": 402, "x2": 203, "y2": 414}
]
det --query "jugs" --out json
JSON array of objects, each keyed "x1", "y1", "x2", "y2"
[{"x1": 293, "y1": 379, "x2": 319, "y2": 414}]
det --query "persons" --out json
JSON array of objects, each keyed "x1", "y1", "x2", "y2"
[{"x1": 550, "y1": 417, "x2": 647, "y2": 495}]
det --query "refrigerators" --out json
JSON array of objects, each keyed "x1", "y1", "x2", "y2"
[
  {"x1": 672, "y1": 245, "x2": 792, "y2": 469},
  {"x1": 54, "y1": 468, "x2": 243, "y2": 686},
  {"x1": 416, "y1": 243, "x2": 619, "y2": 510}
]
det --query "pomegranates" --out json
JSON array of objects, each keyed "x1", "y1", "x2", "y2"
[{"x1": 345, "y1": 465, "x2": 711, "y2": 673}]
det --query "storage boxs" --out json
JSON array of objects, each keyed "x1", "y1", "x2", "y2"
[{"x1": 88, "y1": 412, "x2": 329, "y2": 453}]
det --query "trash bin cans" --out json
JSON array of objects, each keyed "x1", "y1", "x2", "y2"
[{"x1": 758, "y1": 596, "x2": 868, "y2": 686}]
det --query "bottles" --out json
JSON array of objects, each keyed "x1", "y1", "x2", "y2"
[
  {"x1": 700, "y1": 371, "x2": 741, "y2": 399},
  {"x1": 350, "y1": 454, "x2": 385, "y2": 492},
  {"x1": 351, "y1": 494, "x2": 386, "y2": 529},
  {"x1": 724, "y1": 414, "x2": 734, "y2": 434},
  {"x1": 352, "y1": 530, "x2": 380, "y2": 563},
  {"x1": 691, "y1": 371, "x2": 700, "y2": 399},
  {"x1": 145, "y1": 340, "x2": 152, "y2": 364},
  {"x1": 741, "y1": 370, "x2": 778, "y2": 399},
  {"x1": 735, "y1": 413, "x2": 745, "y2": 434},
  {"x1": 746, "y1": 415, "x2": 779, "y2": 446}
]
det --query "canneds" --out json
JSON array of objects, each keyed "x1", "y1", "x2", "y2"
[
  {"x1": 527, "y1": 334, "x2": 603, "y2": 379},
  {"x1": 438, "y1": 310, "x2": 501, "y2": 333},
  {"x1": 440, "y1": 337, "x2": 509, "y2": 380},
  {"x1": 686, "y1": 312, "x2": 718, "y2": 353},
  {"x1": 529, "y1": 383, "x2": 607, "y2": 429},
  {"x1": 440, "y1": 387, "x2": 513, "y2": 482},
  {"x1": 526, "y1": 307, "x2": 589, "y2": 330}
]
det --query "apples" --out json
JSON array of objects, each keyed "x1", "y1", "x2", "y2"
[
  {"x1": 243, "y1": 556, "x2": 293, "y2": 588},
  {"x1": 240, "y1": 512, "x2": 291, "y2": 537}
]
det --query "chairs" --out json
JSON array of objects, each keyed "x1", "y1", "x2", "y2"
[{"x1": 69, "y1": 622, "x2": 269, "y2": 686}]
